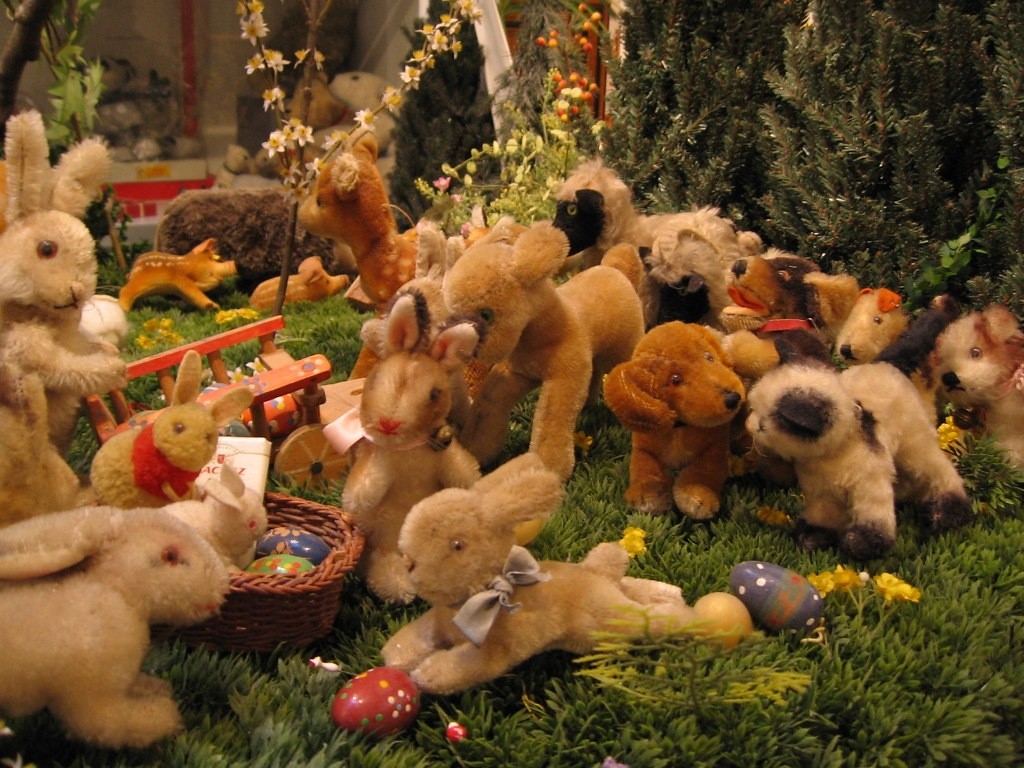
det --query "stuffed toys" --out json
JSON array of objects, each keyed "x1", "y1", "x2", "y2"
[
  {"x1": 0, "y1": 502, "x2": 229, "y2": 755},
  {"x1": 0, "y1": 70, "x2": 1024, "y2": 693}
]
